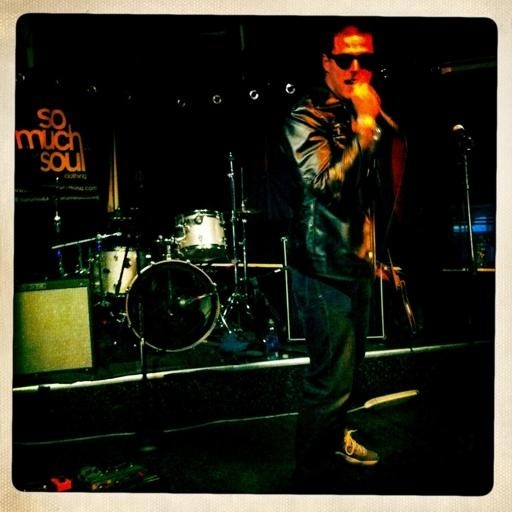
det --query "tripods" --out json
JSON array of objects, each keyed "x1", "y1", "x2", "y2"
[{"x1": 222, "y1": 150, "x2": 287, "y2": 333}]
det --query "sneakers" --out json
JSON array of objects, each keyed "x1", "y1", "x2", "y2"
[{"x1": 335, "y1": 433, "x2": 380, "y2": 466}]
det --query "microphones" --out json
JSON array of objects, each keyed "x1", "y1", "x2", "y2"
[
  {"x1": 379, "y1": 109, "x2": 400, "y2": 131},
  {"x1": 176, "y1": 295, "x2": 196, "y2": 310},
  {"x1": 452, "y1": 124, "x2": 474, "y2": 147},
  {"x1": 112, "y1": 232, "x2": 123, "y2": 237}
]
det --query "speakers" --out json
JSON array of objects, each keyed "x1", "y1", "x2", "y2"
[{"x1": 14, "y1": 278, "x2": 96, "y2": 376}]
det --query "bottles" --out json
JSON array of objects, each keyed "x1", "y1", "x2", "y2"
[{"x1": 266, "y1": 320, "x2": 279, "y2": 360}]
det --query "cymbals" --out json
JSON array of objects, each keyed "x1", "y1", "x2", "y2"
[{"x1": 230, "y1": 208, "x2": 263, "y2": 214}]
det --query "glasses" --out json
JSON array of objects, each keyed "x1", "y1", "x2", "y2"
[{"x1": 327, "y1": 51, "x2": 375, "y2": 71}]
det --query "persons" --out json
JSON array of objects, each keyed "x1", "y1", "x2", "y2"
[{"x1": 280, "y1": 20, "x2": 412, "y2": 479}]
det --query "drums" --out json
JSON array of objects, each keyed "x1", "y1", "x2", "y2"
[
  {"x1": 174, "y1": 208, "x2": 229, "y2": 263},
  {"x1": 88, "y1": 236, "x2": 139, "y2": 298},
  {"x1": 126, "y1": 259, "x2": 220, "y2": 353},
  {"x1": 223, "y1": 291, "x2": 282, "y2": 343}
]
